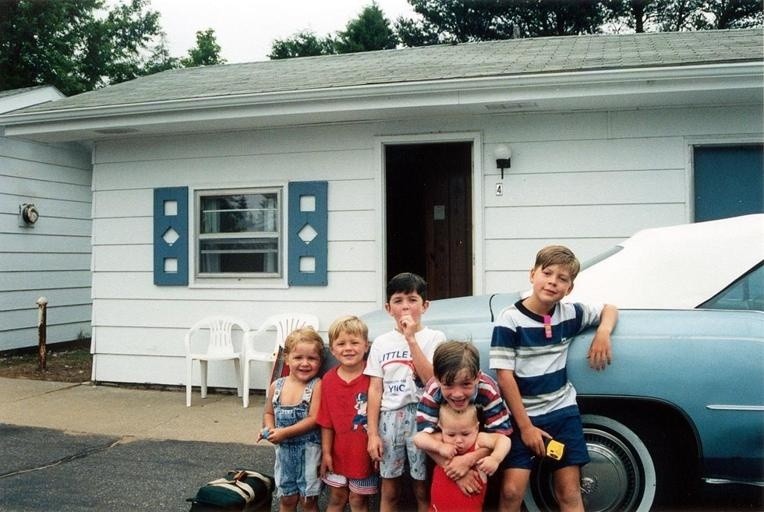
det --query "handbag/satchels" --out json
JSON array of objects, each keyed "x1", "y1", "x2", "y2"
[{"x1": 189, "y1": 469, "x2": 275, "y2": 512}]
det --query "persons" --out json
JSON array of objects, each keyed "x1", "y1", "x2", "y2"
[
  {"x1": 414, "y1": 399, "x2": 512, "y2": 512},
  {"x1": 415, "y1": 340, "x2": 516, "y2": 497},
  {"x1": 489, "y1": 244, "x2": 619, "y2": 512},
  {"x1": 363, "y1": 272, "x2": 448, "y2": 512},
  {"x1": 315, "y1": 314, "x2": 380, "y2": 512},
  {"x1": 256, "y1": 327, "x2": 325, "y2": 512}
]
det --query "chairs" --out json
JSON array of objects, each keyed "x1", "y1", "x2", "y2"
[
  {"x1": 242, "y1": 312, "x2": 320, "y2": 409},
  {"x1": 185, "y1": 315, "x2": 252, "y2": 409}
]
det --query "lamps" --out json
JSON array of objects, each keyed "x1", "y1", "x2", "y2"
[{"x1": 493, "y1": 142, "x2": 512, "y2": 180}]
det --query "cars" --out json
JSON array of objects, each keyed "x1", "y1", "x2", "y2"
[{"x1": 301, "y1": 212, "x2": 764, "y2": 510}]
